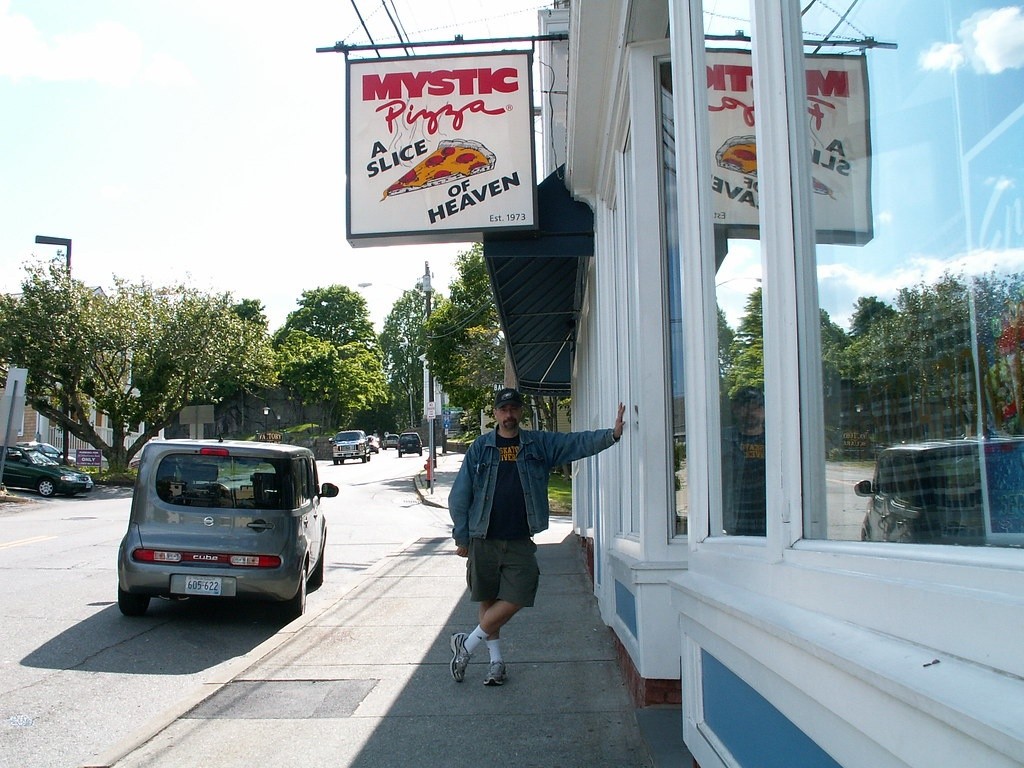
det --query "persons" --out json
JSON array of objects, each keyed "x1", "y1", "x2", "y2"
[
  {"x1": 448, "y1": 388, "x2": 625, "y2": 687},
  {"x1": 721, "y1": 385, "x2": 768, "y2": 537}
]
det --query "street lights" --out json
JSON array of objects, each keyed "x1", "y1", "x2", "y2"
[{"x1": 261, "y1": 406, "x2": 271, "y2": 439}]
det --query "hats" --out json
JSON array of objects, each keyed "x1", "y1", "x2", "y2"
[{"x1": 493, "y1": 388, "x2": 522, "y2": 408}]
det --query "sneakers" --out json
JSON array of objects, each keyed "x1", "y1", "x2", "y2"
[
  {"x1": 450, "y1": 632, "x2": 472, "y2": 682},
  {"x1": 484, "y1": 660, "x2": 509, "y2": 685}
]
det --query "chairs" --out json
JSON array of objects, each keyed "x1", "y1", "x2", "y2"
[{"x1": 192, "y1": 463, "x2": 218, "y2": 490}]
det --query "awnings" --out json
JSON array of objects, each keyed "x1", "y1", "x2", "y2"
[{"x1": 482, "y1": 162, "x2": 595, "y2": 397}]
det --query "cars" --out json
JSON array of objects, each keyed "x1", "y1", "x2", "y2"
[
  {"x1": 365, "y1": 433, "x2": 401, "y2": 454},
  {"x1": 114, "y1": 438, "x2": 339, "y2": 620},
  {"x1": 853, "y1": 435, "x2": 1024, "y2": 549},
  {"x1": 0, "y1": 442, "x2": 94, "y2": 498},
  {"x1": 330, "y1": 429, "x2": 371, "y2": 466},
  {"x1": 397, "y1": 431, "x2": 423, "y2": 458}
]
